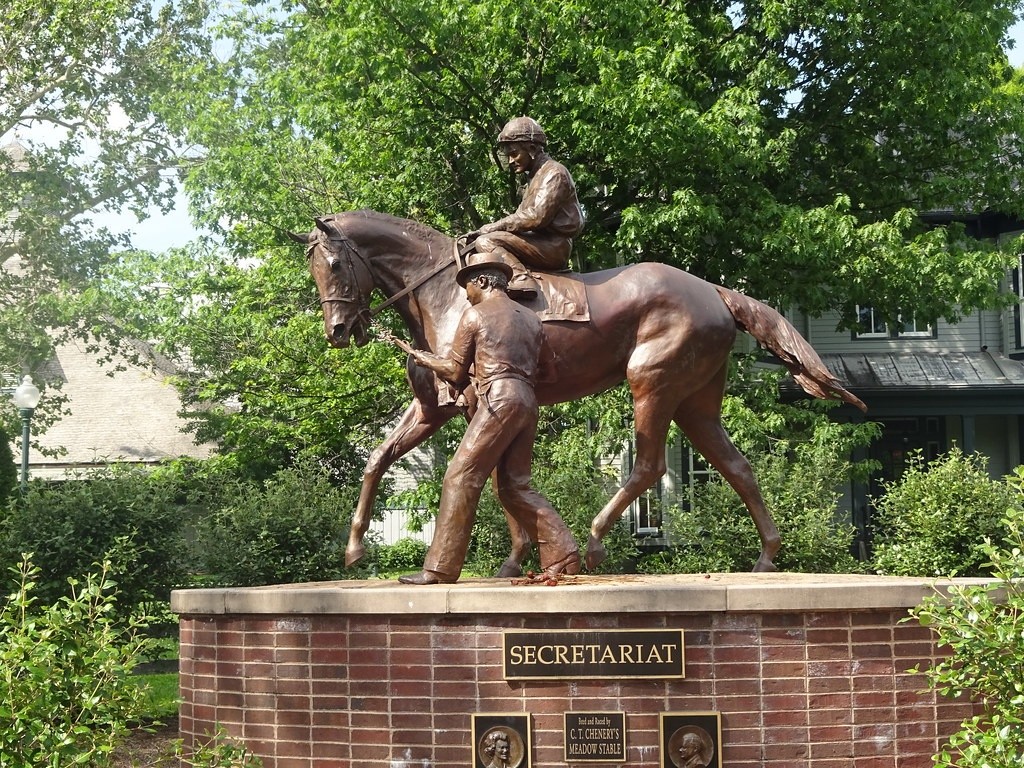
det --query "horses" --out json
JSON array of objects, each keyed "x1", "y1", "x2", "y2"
[{"x1": 284, "y1": 208, "x2": 868, "y2": 574}]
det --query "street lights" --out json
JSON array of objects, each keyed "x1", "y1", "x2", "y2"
[{"x1": 12, "y1": 375, "x2": 44, "y2": 503}]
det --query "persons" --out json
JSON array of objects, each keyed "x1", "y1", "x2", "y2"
[
  {"x1": 397, "y1": 253, "x2": 580, "y2": 585},
  {"x1": 475, "y1": 117, "x2": 584, "y2": 302},
  {"x1": 484, "y1": 731, "x2": 509, "y2": 768},
  {"x1": 679, "y1": 733, "x2": 705, "y2": 768}
]
